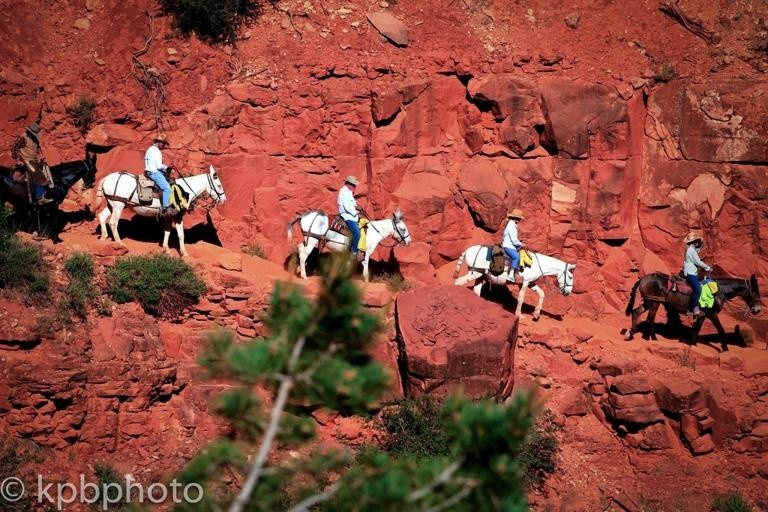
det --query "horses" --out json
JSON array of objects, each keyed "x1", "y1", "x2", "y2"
[
  {"x1": 89, "y1": 164, "x2": 227, "y2": 259},
  {"x1": 625, "y1": 272, "x2": 762, "y2": 352},
  {"x1": 287, "y1": 207, "x2": 412, "y2": 283},
  {"x1": 0, "y1": 149, "x2": 97, "y2": 244},
  {"x1": 453, "y1": 244, "x2": 576, "y2": 320}
]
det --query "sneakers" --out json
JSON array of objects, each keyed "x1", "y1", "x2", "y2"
[
  {"x1": 39, "y1": 196, "x2": 53, "y2": 204},
  {"x1": 687, "y1": 309, "x2": 704, "y2": 316},
  {"x1": 162, "y1": 207, "x2": 176, "y2": 214},
  {"x1": 508, "y1": 276, "x2": 515, "y2": 282}
]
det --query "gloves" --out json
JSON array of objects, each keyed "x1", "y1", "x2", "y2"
[{"x1": 166, "y1": 166, "x2": 174, "y2": 173}]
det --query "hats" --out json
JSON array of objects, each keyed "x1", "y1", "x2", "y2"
[
  {"x1": 508, "y1": 209, "x2": 524, "y2": 220},
  {"x1": 152, "y1": 133, "x2": 170, "y2": 145},
  {"x1": 25, "y1": 123, "x2": 41, "y2": 137},
  {"x1": 345, "y1": 176, "x2": 359, "y2": 186},
  {"x1": 685, "y1": 233, "x2": 700, "y2": 244}
]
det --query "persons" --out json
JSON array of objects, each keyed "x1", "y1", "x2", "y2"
[
  {"x1": 502, "y1": 208, "x2": 528, "y2": 284},
  {"x1": 680, "y1": 231, "x2": 714, "y2": 317},
  {"x1": 336, "y1": 175, "x2": 363, "y2": 255},
  {"x1": 144, "y1": 132, "x2": 174, "y2": 213},
  {"x1": 11, "y1": 121, "x2": 53, "y2": 206}
]
